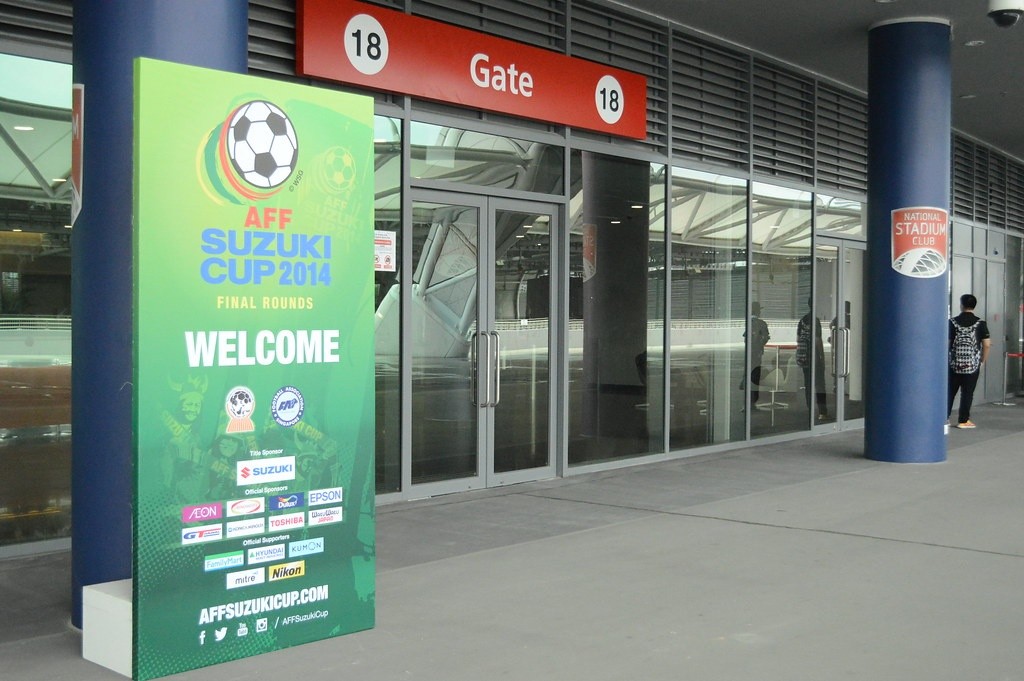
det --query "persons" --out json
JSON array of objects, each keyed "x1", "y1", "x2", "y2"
[
  {"x1": 739, "y1": 302, "x2": 770, "y2": 412},
  {"x1": 947, "y1": 294, "x2": 991, "y2": 429},
  {"x1": 797, "y1": 295, "x2": 836, "y2": 422},
  {"x1": 830, "y1": 300, "x2": 851, "y2": 329}
]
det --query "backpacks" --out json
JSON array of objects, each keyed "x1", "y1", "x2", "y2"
[
  {"x1": 795, "y1": 320, "x2": 812, "y2": 367},
  {"x1": 950, "y1": 318, "x2": 983, "y2": 374}
]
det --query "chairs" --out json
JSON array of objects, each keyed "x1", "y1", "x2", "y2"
[{"x1": 752, "y1": 353, "x2": 793, "y2": 411}]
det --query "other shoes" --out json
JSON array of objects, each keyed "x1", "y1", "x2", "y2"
[
  {"x1": 818, "y1": 414, "x2": 835, "y2": 419},
  {"x1": 957, "y1": 420, "x2": 976, "y2": 428}
]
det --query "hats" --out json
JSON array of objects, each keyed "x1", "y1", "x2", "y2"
[{"x1": 752, "y1": 302, "x2": 764, "y2": 310}]
